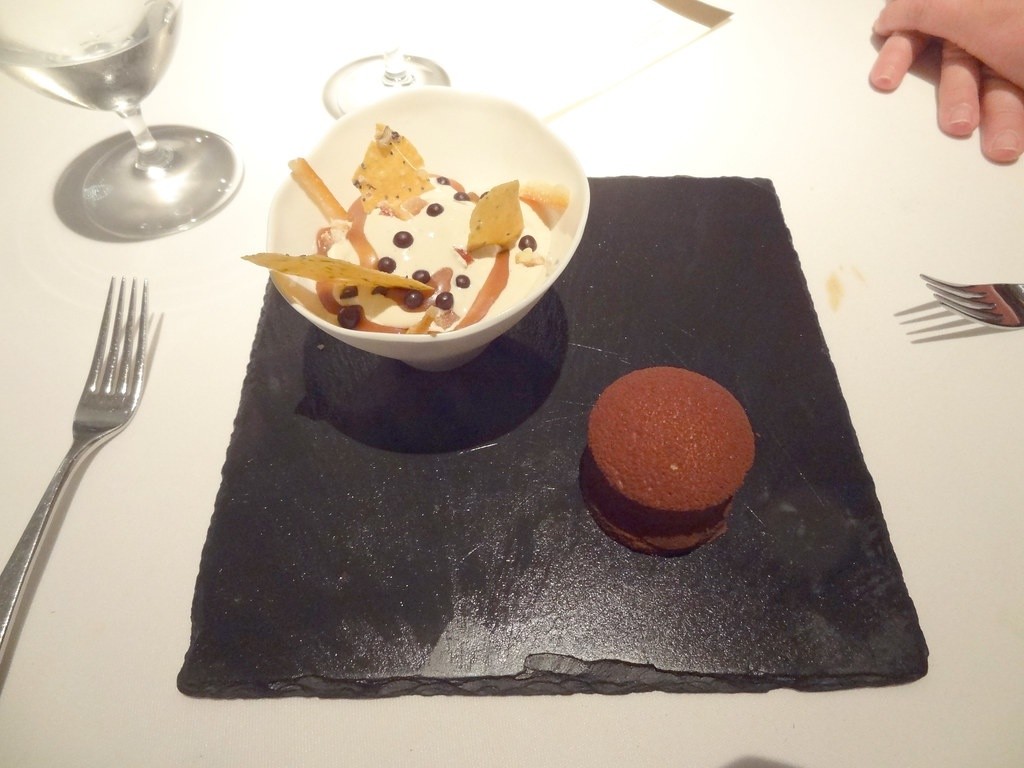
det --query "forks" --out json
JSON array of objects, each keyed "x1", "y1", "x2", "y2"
[{"x1": 0, "y1": 275, "x2": 151, "y2": 682}]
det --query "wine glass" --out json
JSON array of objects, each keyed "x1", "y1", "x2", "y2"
[
  {"x1": 323, "y1": 0, "x2": 450, "y2": 121},
  {"x1": 0, "y1": 0, "x2": 243, "y2": 245}
]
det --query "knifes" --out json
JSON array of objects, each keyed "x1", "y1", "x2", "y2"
[{"x1": 918, "y1": 274, "x2": 1024, "y2": 328}]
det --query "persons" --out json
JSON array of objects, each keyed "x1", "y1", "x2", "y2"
[{"x1": 868, "y1": 0, "x2": 1024, "y2": 163}]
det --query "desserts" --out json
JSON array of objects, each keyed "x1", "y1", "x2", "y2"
[
  {"x1": 586, "y1": 365, "x2": 755, "y2": 554},
  {"x1": 240, "y1": 124, "x2": 571, "y2": 334}
]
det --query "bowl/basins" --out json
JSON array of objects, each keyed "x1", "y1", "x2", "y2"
[{"x1": 265, "y1": 86, "x2": 592, "y2": 372}]
